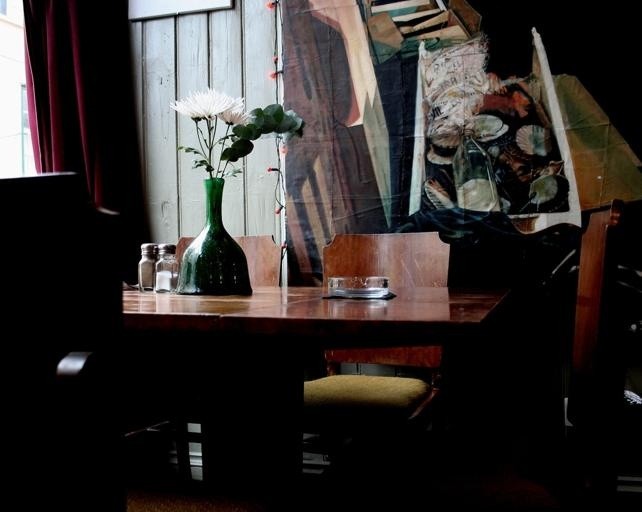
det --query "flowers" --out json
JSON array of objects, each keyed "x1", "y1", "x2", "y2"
[{"x1": 165, "y1": 80, "x2": 306, "y2": 184}]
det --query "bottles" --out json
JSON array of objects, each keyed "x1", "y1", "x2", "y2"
[
  {"x1": 451, "y1": 129, "x2": 500, "y2": 214},
  {"x1": 138, "y1": 242, "x2": 179, "y2": 293},
  {"x1": 138, "y1": 294, "x2": 172, "y2": 314}
]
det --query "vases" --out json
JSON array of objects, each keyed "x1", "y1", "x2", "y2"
[{"x1": 175, "y1": 178, "x2": 256, "y2": 297}]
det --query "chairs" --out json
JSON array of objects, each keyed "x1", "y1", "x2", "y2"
[
  {"x1": 172, "y1": 235, "x2": 283, "y2": 287},
  {"x1": 304, "y1": 230, "x2": 453, "y2": 511},
  {"x1": 0, "y1": 170, "x2": 172, "y2": 512}
]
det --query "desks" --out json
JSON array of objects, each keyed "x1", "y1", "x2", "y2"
[{"x1": 123, "y1": 286, "x2": 514, "y2": 512}]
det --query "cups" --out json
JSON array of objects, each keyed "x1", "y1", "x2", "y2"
[{"x1": 327, "y1": 276, "x2": 389, "y2": 298}]
